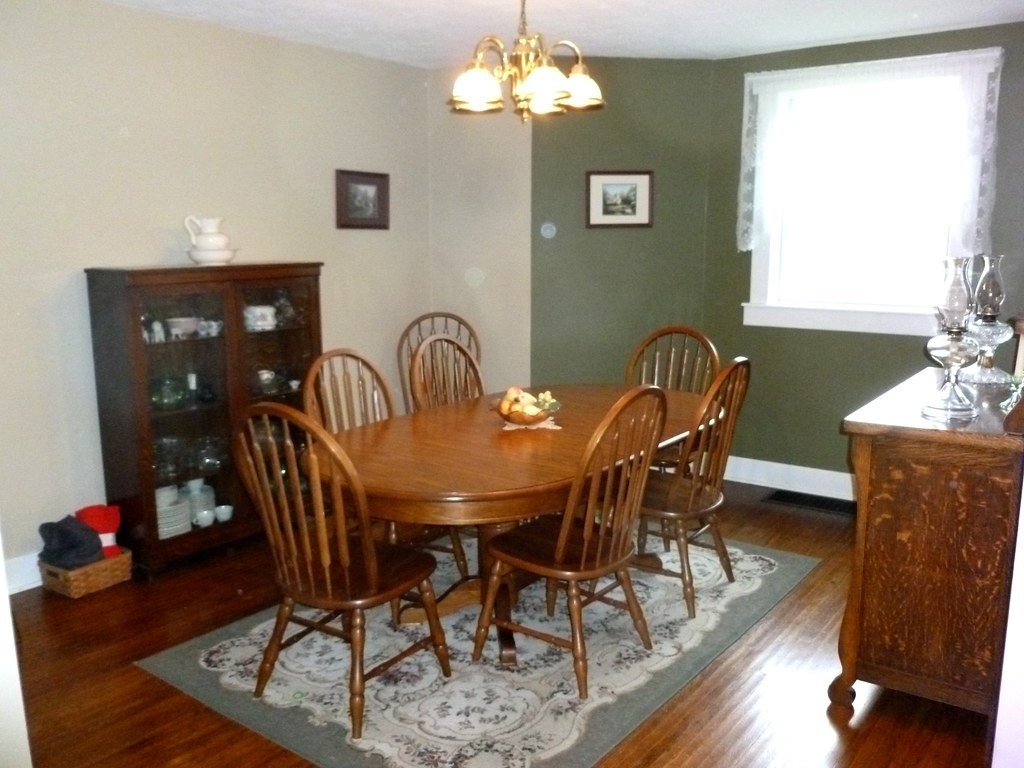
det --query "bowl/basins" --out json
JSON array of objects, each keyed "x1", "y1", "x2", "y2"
[
  {"x1": 187, "y1": 249, "x2": 239, "y2": 267},
  {"x1": 156, "y1": 484, "x2": 178, "y2": 507},
  {"x1": 487, "y1": 397, "x2": 564, "y2": 426}
]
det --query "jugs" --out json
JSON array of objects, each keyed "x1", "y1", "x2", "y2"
[{"x1": 185, "y1": 214, "x2": 230, "y2": 250}]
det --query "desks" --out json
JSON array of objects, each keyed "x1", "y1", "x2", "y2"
[{"x1": 305, "y1": 383, "x2": 727, "y2": 671}]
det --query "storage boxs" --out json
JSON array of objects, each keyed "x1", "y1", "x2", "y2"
[{"x1": 37, "y1": 545, "x2": 130, "y2": 597}]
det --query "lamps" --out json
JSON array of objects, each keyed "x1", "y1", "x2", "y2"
[
  {"x1": 450, "y1": 1, "x2": 605, "y2": 123},
  {"x1": 924, "y1": 252, "x2": 1019, "y2": 419}
]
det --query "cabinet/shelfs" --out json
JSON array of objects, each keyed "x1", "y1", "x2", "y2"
[
  {"x1": 826, "y1": 362, "x2": 1024, "y2": 723},
  {"x1": 83, "y1": 260, "x2": 323, "y2": 577}
]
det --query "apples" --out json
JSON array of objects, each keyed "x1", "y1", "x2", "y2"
[{"x1": 498, "y1": 386, "x2": 541, "y2": 422}]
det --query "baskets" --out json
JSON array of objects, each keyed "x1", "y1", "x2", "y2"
[{"x1": 38, "y1": 547, "x2": 131, "y2": 599}]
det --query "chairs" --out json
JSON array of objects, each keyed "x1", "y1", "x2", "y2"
[
  {"x1": 471, "y1": 385, "x2": 669, "y2": 701},
  {"x1": 229, "y1": 403, "x2": 451, "y2": 740},
  {"x1": 581, "y1": 356, "x2": 752, "y2": 619},
  {"x1": 307, "y1": 314, "x2": 485, "y2": 625},
  {"x1": 602, "y1": 327, "x2": 723, "y2": 554}
]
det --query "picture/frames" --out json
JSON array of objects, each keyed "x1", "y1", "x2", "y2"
[
  {"x1": 586, "y1": 169, "x2": 653, "y2": 229},
  {"x1": 335, "y1": 169, "x2": 390, "y2": 229}
]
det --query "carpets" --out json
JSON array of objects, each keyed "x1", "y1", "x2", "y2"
[{"x1": 133, "y1": 500, "x2": 822, "y2": 767}]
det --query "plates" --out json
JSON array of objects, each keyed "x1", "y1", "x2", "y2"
[{"x1": 156, "y1": 485, "x2": 216, "y2": 540}]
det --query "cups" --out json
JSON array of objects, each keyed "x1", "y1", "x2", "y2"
[
  {"x1": 196, "y1": 318, "x2": 223, "y2": 337},
  {"x1": 184, "y1": 479, "x2": 205, "y2": 495},
  {"x1": 193, "y1": 510, "x2": 215, "y2": 527},
  {"x1": 214, "y1": 505, "x2": 233, "y2": 521},
  {"x1": 258, "y1": 370, "x2": 275, "y2": 385}
]
